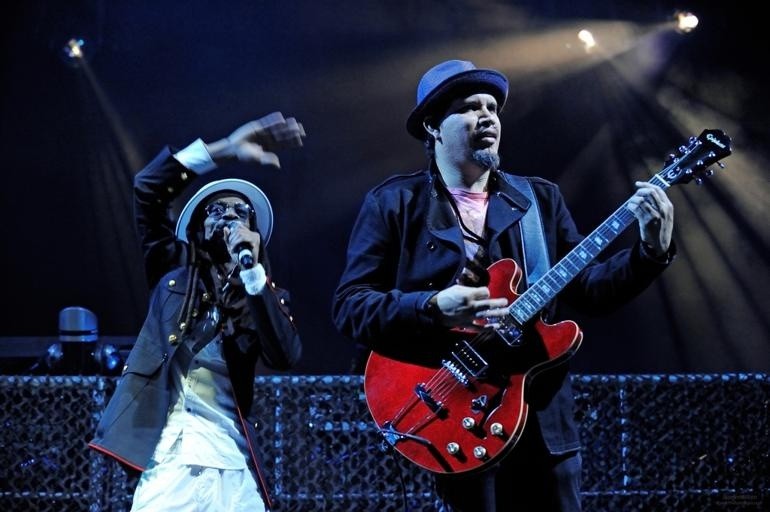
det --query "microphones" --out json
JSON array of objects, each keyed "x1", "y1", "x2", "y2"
[{"x1": 228, "y1": 221, "x2": 253, "y2": 269}]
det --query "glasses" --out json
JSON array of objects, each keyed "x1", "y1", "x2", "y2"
[{"x1": 205, "y1": 202, "x2": 254, "y2": 219}]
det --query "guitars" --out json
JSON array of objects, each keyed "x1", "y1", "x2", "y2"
[{"x1": 363, "y1": 129, "x2": 732, "y2": 479}]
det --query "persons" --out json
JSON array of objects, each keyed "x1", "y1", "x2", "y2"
[
  {"x1": 331, "y1": 59, "x2": 677, "y2": 510},
  {"x1": 85, "y1": 112, "x2": 303, "y2": 511}
]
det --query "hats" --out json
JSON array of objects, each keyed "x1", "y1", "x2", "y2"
[
  {"x1": 175, "y1": 178, "x2": 274, "y2": 249},
  {"x1": 406, "y1": 60, "x2": 508, "y2": 141}
]
